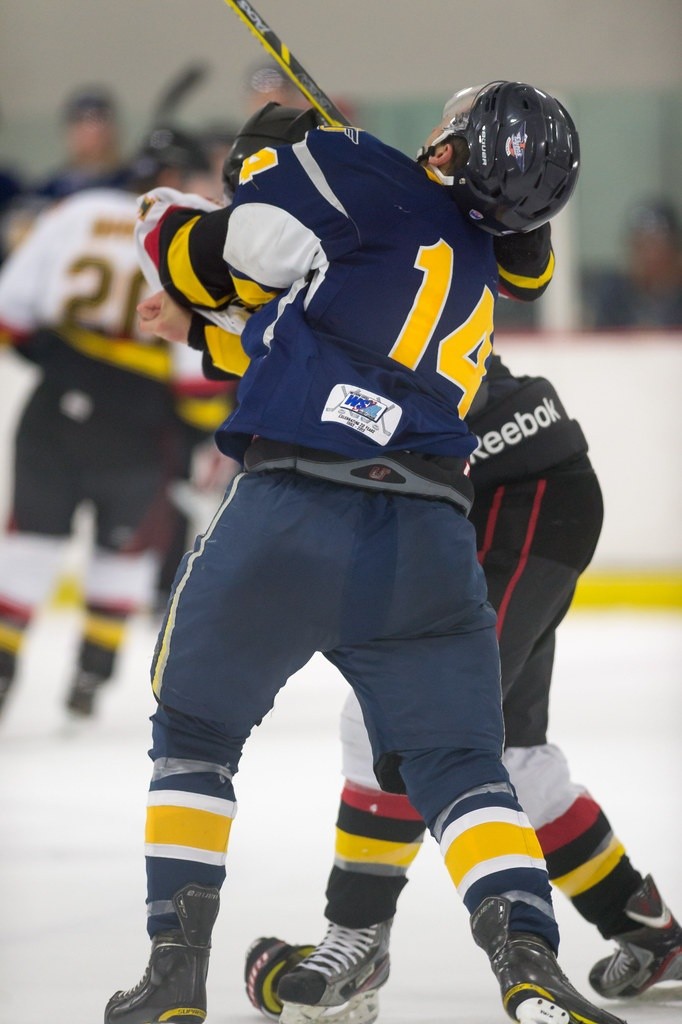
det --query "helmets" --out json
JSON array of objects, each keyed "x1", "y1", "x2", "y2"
[{"x1": 452, "y1": 80, "x2": 581, "y2": 239}]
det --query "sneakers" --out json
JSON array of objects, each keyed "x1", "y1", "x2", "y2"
[
  {"x1": 470, "y1": 897, "x2": 626, "y2": 1024},
  {"x1": 587, "y1": 873, "x2": 681, "y2": 1000},
  {"x1": 276, "y1": 914, "x2": 391, "y2": 1024},
  {"x1": 102, "y1": 884, "x2": 220, "y2": 1024}
]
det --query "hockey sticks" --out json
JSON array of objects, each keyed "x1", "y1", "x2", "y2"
[{"x1": 230, "y1": 1, "x2": 370, "y2": 135}]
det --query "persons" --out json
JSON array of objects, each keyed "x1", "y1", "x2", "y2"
[
  {"x1": 0, "y1": 85, "x2": 240, "y2": 719},
  {"x1": 107, "y1": 81, "x2": 682, "y2": 1024}
]
